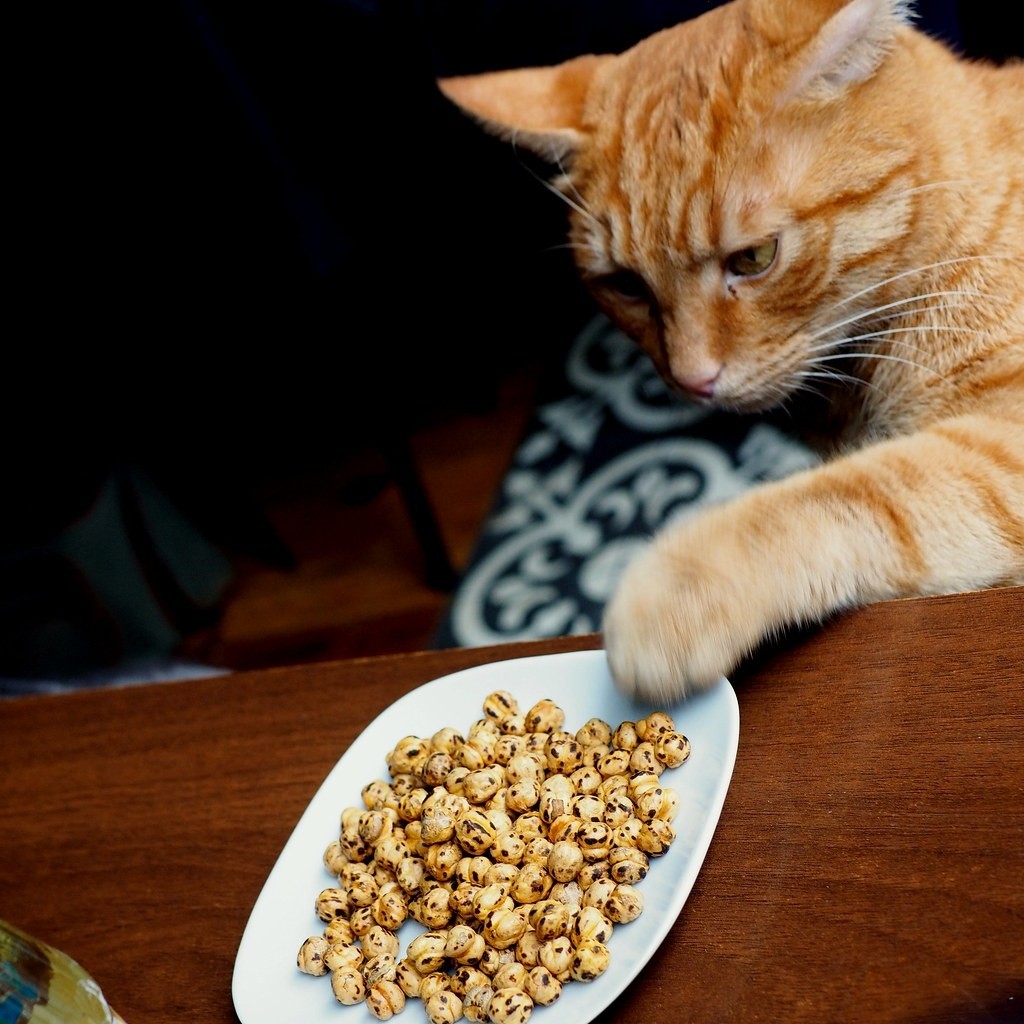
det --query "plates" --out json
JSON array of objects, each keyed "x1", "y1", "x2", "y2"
[{"x1": 231, "y1": 651, "x2": 742, "y2": 1023}]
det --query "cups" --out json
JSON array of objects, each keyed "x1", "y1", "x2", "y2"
[{"x1": 0, "y1": 922, "x2": 128, "y2": 1024}]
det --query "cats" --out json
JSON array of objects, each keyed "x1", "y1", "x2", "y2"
[{"x1": 435, "y1": 0, "x2": 1022, "y2": 703}]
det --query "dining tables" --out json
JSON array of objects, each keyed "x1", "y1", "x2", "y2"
[{"x1": 0, "y1": 590, "x2": 1024, "y2": 1024}]
